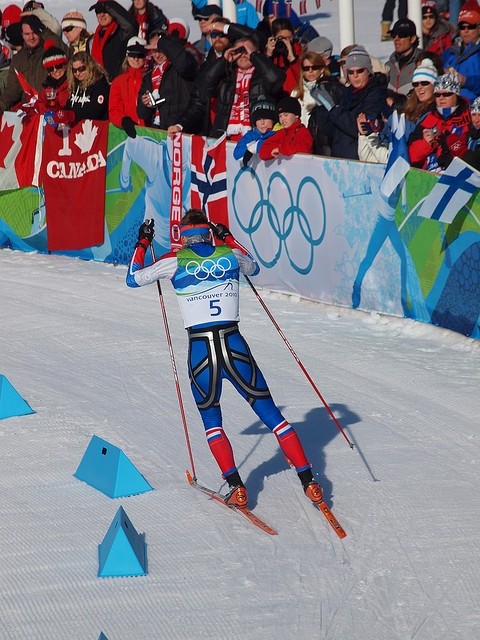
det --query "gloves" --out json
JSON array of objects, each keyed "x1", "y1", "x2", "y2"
[
  {"x1": 24, "y1": 14, "x2": 45, "y2": 36},
  {"x1": 360, "y1": 119, "x2": 384, "y2": 136},
  {"x1": 53, "y1": 110, "x2": 75, "y2": 125},
  {"x1": 89, "y1": 2, "x2": 104, "y2": 11},
  {"x1": 243, "y1": 150, "x2": 253, "y2": 167},
  {"x1": 310, "y1": 85, "x2": 324, "y2": 106},
  {"x1": 122, "y1": 116, "x2": 138, "y2": 138},
  {"x1": 139, "y1": 219, "x2": 154, "y2": 242},
  {"x1": 213, "y1": 223, "x2": 230, "y2": 240},
  {"x1": 312, "y1": 85, "x2": 336, "y2": 112}
]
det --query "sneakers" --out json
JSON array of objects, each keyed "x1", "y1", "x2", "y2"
[
  {"x1": 306, "y1": 483, "x2": 323, "y2": 502},
  {"x1": 224, "y1": 485, "x2": 248, "y2": 506}
]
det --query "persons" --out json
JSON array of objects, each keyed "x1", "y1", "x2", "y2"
[
  {"x1": 352, "y1": 176, "x2": 415, "y2": 319},
  {"x1": 260, "y1": 98, "x2": 314, "y2": 160},
  {"x1": 128, "y1": 0, "x2": 172, "y2": 38},
  {"x1": 126, "y1": 210, "x2": 324, "y2": 507},
  {"x1": 190, "y1": 0, "x2": 259, "y2": 29},
  {"x1": 1, "y1": 5, "x2": 22, "y2": 59},
  {"x1": 137, "y1": 30, "x2": 198, "y2": 129},
  {"x1": 408, "y1": 72, "x2": 470, "y2": 174},
  {"x1": 291, "y1": 52, "x2": 331, "y2": 128},
  {"x1": 310, "y1": 46, "x2": 388, "y2": 160},
  {"x1": 21, "y1": 0, "x2": 61, "y2": 38},
  {"x1": 234, "y1": 95, "x2": 279, "y2": 166},
  {"x1": 335, "y1": 46, "x2": 355, "y2": 87},
  {"x1": 381, "y1": 0, "x2": 408, "y2": 41},
  {"x1": 54, "y1": 51, "x2": 108, "y2": 126},
  {"x1": 62, "y1": 9, "x2": 94, "y2": 55},
  {"x1": 133, "y1": 241, "x2": 147, "y2": 252},
  {"x1": 357, "y1": 89, "x2": 407, "y2": 163},
  {"x1": 398, "y1": 59, "x2": 438, "y2": 142},
  {"x1": 307, "y1": 36, "x2": 336, "y2": 67},
  {"x1": 108, "y1": 36, "x2": 151, "y2": 138},
  {"x1": 439, "y1": 97, "x2": 479, "y2": 172},
  {"x1": 1, "y1": 15, "x2": 73, "y2": 114},
  {"x1": 11, "y1": 40, "x2": 69, "y2": 116},
  {"x1": 192, "y1": 4, "x2": 223, "y2": 61},
  {"x1": 198, "y1": 37, "x2": 286, "y2": 141},
  {"x1": 168, "y1": 18, "x2": 260, "y2": 137},
  {"x1": 119, "y1": 135, "x2": 191, "y2": 266},
  {"x1": 265, "y1": 19, "x2": 304, "y2": 95},
  {"x1": 421, "y1": 0, "x2": 453, "y2": 56},
  {"x1": 385, "y1": 20, "x2": 444, "y2": 101},
  {"x1": 442, "y1": 0, "x2": 480, "y2": 104}
]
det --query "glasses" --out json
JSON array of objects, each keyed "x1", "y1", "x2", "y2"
[
  {"x1": 128, "y1": 53, "x2": 145, "y2": 58},
  {"x1": 412, "y1": 81, "x2": 430, "y2": 87},
  {"x1": 63, "y1": 26, "x2": 74, "y2": 32},
  {"x1": 303, "y1": 65, "x2": 323, "y2": 71},
  {"x1": 391, "y1": 32, "x2": 413, "y2": 38},
  {"x1": 210, "y1": 32, "x2": 226, "y2": 38},
  {"x1": 347, "y1": 68, "x2": 366, "y2": 74},
  {"x1": 459, "y1": 24, "x2": 480, "y2": 30},
  {"x1": 434, "y1": 92, "x2": 454, "y2": 96},
  {"x1": 198, "y1": 18, "x2": 209, "y2": 21},
  {"x1": 72, "y1": 66, "x2": 86, "y2": 73},
  {"x1": 48, "y1": 63, "x2": 63, "y2": 72}
]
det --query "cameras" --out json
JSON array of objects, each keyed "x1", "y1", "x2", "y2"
[
  {"x1": 428, "y1": 127, "x2": 439, "y2": 137},
  {"x1": 141, "y1": 91, "x2": 166, "y2": 107},
  {"x1": 273, "y1": 38, "x2": 286, "y2": 51}
]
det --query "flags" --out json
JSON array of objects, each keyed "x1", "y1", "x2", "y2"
[
  {"x1": 87, "y1": 0, "x2": 139, "y2": 82},
  {"x1": 191, "y1": 134, "x2": 229, "y2": 246},
  {"x1": 417, "y1": 157, "x2": 480, "y2": 224},
  {"x1": 44, "y1": 120, "x2": 111, "y2": 251},
  {"x1": 0, "y1": 111, "x2": 44, "y2": 191},
  {"x1": 380, "y1": 115, "x2": 411, "y2": 198},
  {"x1": 389, "y1": 109, "x2": 398, "y2": 154}
]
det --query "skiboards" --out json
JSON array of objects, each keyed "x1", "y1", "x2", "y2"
[{"x1": 184, "y1": 459, "x2": 347, "y2": 539}]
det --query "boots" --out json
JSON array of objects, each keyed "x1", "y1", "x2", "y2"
[{"x1": 381, "y1": 21, "x2": 393, "y2": 41}]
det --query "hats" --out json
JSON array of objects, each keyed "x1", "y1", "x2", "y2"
[
  {"x1": 61, "y1": 9, "x2": 87, "y2": 31},
  {"x1": 127, "y1": 37, "x2": 147, "y2": 53},
  {"x1": 1, "y1": 5, "x2": 23, "y2": 28},
  {"x1": 387, "y1": 19, "x2": 416, "y2": 35},
  {"x1": 277, "y1": 97, "x2": 301, "y2": 118},
  {"x1": 470, "y1": 96, "x2": 480, "y2": 114},
  {"x1": 422, "y1": 15, "x2": 435, "y2": 19},
  {"x1": 346, "y1": 45, "x2": 375, "y2": 78},
  {"x1": 250, "y1": 95, "x2": 279, "y2": 127},
  {"x1": 168, "y1": 18, "x2": 189, "y2": 40},
  {"x1": 5, "y1": 23, "x2": 24, "y2": 46},
  {"x1": 308, "y1": 36, "x2": 333, "y2": 54},
  {"x1": 422, "y1": 0, "x2": 436, "y2": 15},
  {"x1": 194, "y1": 5, "x2": 223, "y2": 20},
  {"x1": 457, "y1": 10, "x2": 480, "y2": 24},
  {"x1": 41, "y1": 40, "x2": 68, "y2": 70},
  {"x1": 22, "y1": 0, "x2": 44, "y2": 12},
  {"x1": 434, "y1": 67, "x2": 461, "y2": 96},
  {"x1": 412, "y1": 58, "x2": 438, "y2": 85}
]
